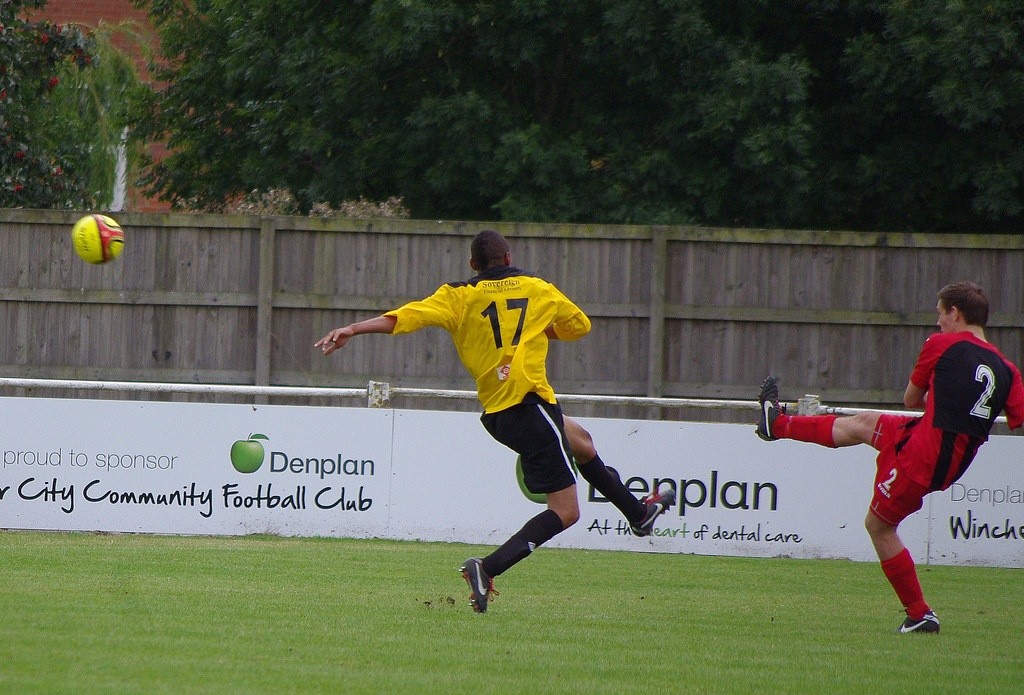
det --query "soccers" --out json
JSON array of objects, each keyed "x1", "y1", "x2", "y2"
[{"x1": 71, "y1": 211, "x2": 125, "y2": 266}]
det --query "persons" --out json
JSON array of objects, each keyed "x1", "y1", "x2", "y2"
[
  {"x1": 754, "y1": 283, "x2": 1024, "y2": 634},
  {"x1": 313, "y1": 230, "x2": 674, "y2": 613}
]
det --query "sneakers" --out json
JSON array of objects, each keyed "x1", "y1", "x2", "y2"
[
  {"x1": 754, "y1": 374, "x2": 787, "y2": 443},
  {"x1": 631, "y1": 489, "x2": 674, "y2": 537},
  {"x1": 459, "y1": 556, "x2": 499, "y2": 613},
  {"x1": 896, "y1": 609, "x2": 940, "y2": 636}
]
